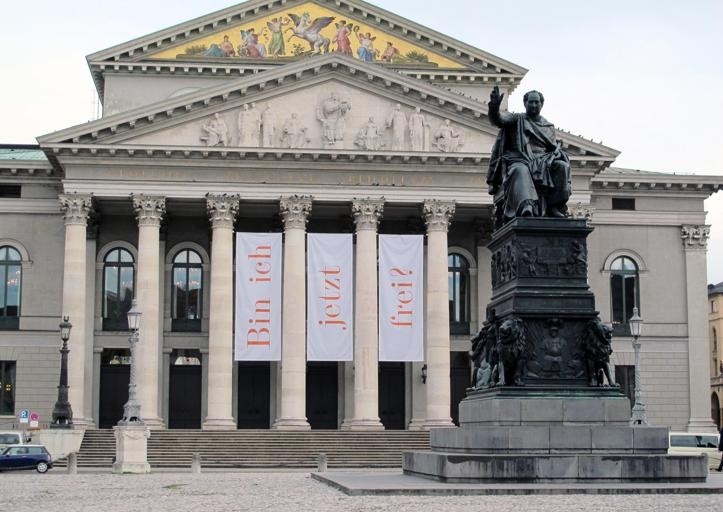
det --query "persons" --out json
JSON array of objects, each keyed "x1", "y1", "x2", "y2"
[
  {"x1": 200, "y1": 92, "x2": 466, "y2": 153},
  {"x1": 532, "y1": 327, "x2": 576, "y2": 378},
  {"x1": 207, "y1": 17, "x2": 399, "y2": 63},
  {"x1": 487, "y1": 84, "x2": 573, "y2": 229}
]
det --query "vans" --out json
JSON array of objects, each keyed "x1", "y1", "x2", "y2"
[{"x1": 668, "y1": 432, "x2": 722, "y2": 470}]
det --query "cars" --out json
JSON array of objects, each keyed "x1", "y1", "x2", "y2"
[{"x1": 0, "y1": 430, "x2": 53, "y2": 474}]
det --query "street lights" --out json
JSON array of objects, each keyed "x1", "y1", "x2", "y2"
[
  {"x1": 116, "y1": 299, "x2": 144, "y2": 425},
  {"x1": 51, "y1": 315, "x2": 73, "y2": 426},
  {"x1": 630, "y1": 307, "x2": 650, "y2": 426}
]
